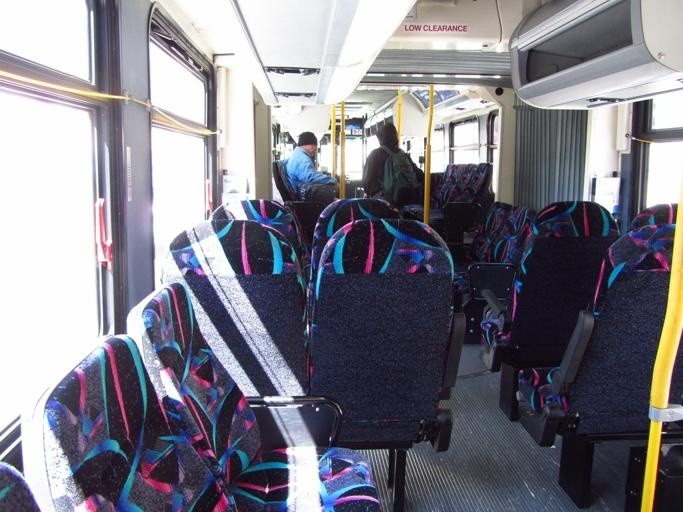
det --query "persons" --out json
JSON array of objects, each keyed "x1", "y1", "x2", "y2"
[
  {"x1": 286, "y1": 130, "x2": 341, "y2": 201},
  {"x1": 361, "y1": 123, "x2": 424, "y2": 205}
]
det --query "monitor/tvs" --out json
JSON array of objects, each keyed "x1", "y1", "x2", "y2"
[{"x1": 344, "y1": 119, "x2": 362, "y2": 136}]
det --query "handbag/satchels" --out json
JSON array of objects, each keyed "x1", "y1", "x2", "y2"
[{"x1": 300, "y1": 181, "x2": 334, "y2": 202}]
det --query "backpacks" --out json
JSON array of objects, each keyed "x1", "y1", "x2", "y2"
[{"x1": 381, "y1": 144, "x2": 418, "y2": 209}]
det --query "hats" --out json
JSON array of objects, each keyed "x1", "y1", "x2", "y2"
[{"x1": 298, "y1": 131, "x2": 317, "y2": 146}]
